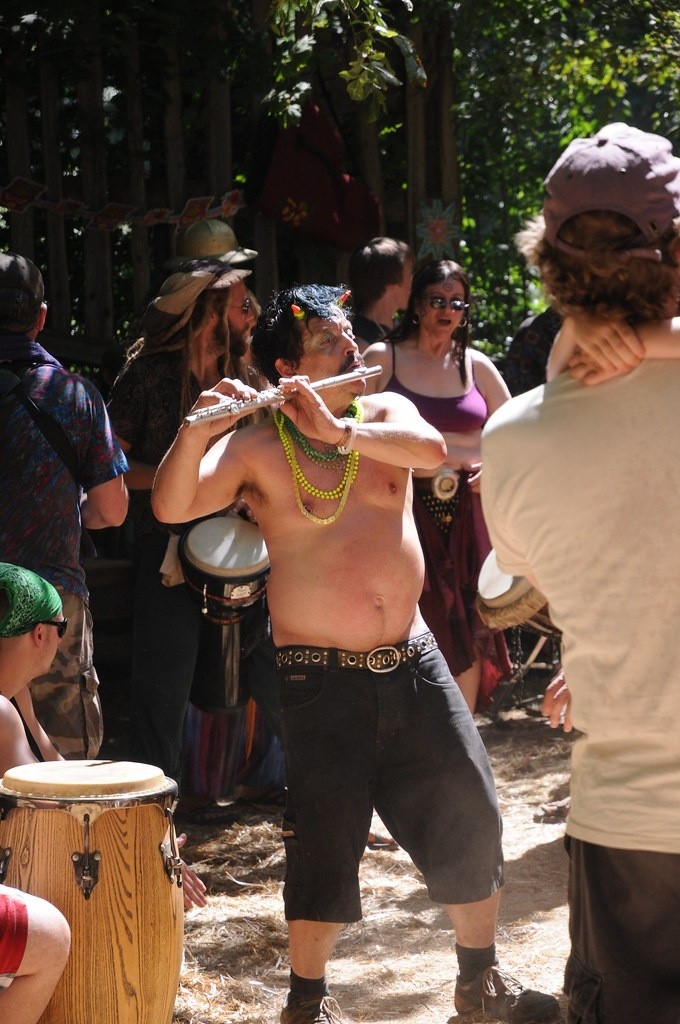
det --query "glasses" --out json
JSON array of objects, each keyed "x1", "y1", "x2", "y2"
[
  {"x1": 223, "y1": 298, "x2": 250, "y2": 315},
  {"x1": 33, "y1": 618, "x2": 68, "y2": 637},
  {"x1": 419, "y1": 296, "x2": 470, "y2": 311}
]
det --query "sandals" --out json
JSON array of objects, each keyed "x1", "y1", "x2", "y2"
[{"x1": 252, "y1": 788, "x2": 287, "y2": 813}]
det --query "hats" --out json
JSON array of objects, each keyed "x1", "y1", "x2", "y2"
[
  {"x1": 539, "y1": 123, "x2": 680, "y2": 259},
  {"x1": 162, "y1": 220, "x2": 258, "y2": 270},
  {"x1": 204, "y1": 266, "x2": 253, "y2": 289},
  {"x1": 0, "y1": 252, "x2": 44, "y2": 304}
]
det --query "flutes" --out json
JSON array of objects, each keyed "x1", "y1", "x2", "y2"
[{"x1": 182, "y1": 364, "x2": 383, "y2": 428}]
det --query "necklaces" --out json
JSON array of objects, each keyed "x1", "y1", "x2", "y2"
[{"x1": 271, "y1": 398, "x2": 359, "y2": 526}]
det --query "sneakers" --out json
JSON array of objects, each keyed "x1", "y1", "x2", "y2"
[
  {"x1": 455, "y1": 961, "x2": 558, "y2": 1019},
  {"x1": 280, "y1": 994, "x2": 344, "y2": 1024}
]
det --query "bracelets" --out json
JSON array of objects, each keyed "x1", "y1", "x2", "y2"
[
  {"x1": 338, "y1": 414, "x2": 358, "y2": 457},
  {"x1": 334, "y1": 417, "x2": 350, "y2": 449}
]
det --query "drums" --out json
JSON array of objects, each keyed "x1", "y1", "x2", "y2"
[
  {"x1": 0, "y1": 759, "x2": 185, "y2": 1024},
  {"x1": 475, "y1": 546, "x2": 562, "y2": 638},
  {"x1": 178, "y1": 513, "x2": 271, "y2": 716}
]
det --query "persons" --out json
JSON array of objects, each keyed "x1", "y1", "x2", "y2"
[
  {"x1": 85, "y1": 268, "x2": 256, "y2": 828},
  {"x1": 171, "y1": 341, "x2": 289, "y2": 827},
  {"x1": 0, "y1": 560, "x2": 208, "y2": 915},
  {"x1": 477, "y1": 120, "x2": 679, "y2": 1024},
  {"x1": 0, "y1": 885, "x2": 73, "y2": 1024},
  {"x1": 152, "y1": 286, "x2": 560, "y2": 1024},
  {"x1": 339, "y1": 236, "x2": 415, "y2": 350},
  {"x1": 359, "y1": 260, "x2": 515, "y2": 720},
  {"x1": 0, "y1": 251, "x2": 129, "y2": 764}
]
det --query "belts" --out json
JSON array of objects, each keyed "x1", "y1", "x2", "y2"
[{"x1": 276, "y1": 632, "x2": 438, "y2": 673}]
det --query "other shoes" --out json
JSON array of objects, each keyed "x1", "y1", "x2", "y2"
[{"x1": 190, "y1": 802, "x2": 240, "y2": 822}]
